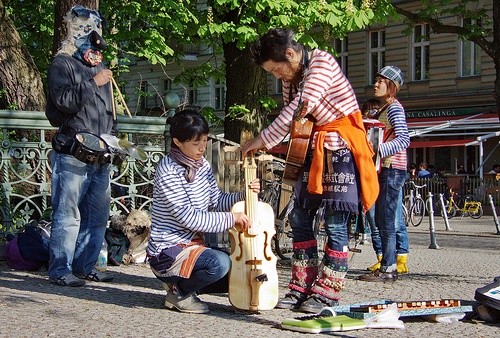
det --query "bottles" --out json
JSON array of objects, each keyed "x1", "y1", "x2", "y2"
[{"x1": 95, "y1": 236, "x2": 108, "y2": 272}]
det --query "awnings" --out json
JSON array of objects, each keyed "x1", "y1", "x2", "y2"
[{"x1": 405, "y1": 113, "x2": 500, "y2": 188}]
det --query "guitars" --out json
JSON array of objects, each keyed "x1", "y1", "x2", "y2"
[
  {"x1": 227, "y1": 129, "x2": 280, "y2": 313},
  {"x1": 282, "y1": 100, "x2": 314, "y2": 182}
]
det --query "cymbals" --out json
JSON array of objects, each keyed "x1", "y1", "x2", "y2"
[{"x1": 118, "y1": 139, "x2": 147, "y2": 160}]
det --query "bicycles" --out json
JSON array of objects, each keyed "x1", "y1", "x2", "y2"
[
  {"x1": 401, "y1": 203, "x2": 409, "y2": 226},
  {"x1": 404, "y1": 181, "x2": 427, "y2": 227},
  {"x1": 262, "y1": 160, "x2": 375, "y2": 266},
  {"x1": 424, "y1": 190, "x2": 479, "y2": 215},
  {"x1": 441, "y1": 187, "x2": 484, "y2": 220}
]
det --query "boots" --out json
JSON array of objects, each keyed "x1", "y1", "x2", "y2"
[
  {"x1": 367, "y1": 254, "x2": 384, "y2": 271},
  {"x1": 396, "y1": 253, "x2": 408, "y2": 273}
]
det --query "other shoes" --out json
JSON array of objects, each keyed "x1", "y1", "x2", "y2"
[
  {"x1": 161, "y1": 279, "x2": 172, "y2": 293},
  {"x1": 276, "y1": 295, "x2": 301, "y2": 309},
  {"x1": 164, "y1": 291, "x2": 209, "y2": 314},
  {"x1": 359, "y1": 268, "x2": 398, "y2": 283},
  {"x1": 299, "y1": 298, "x2": 337, "y2": 313}
]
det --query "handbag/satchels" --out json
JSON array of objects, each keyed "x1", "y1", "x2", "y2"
[
  {"x1": 73, "y1": 226, "x2": 150, "y2": 269},
  {"x1": 5, "y1": 226, "x2": 48, "y2": 272}
]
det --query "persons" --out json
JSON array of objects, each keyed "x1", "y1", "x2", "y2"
[
  {"x1": 404, "y1": 159, "x2": 500, "y2": 197},
  {"x1": 240, "y1": 28, "x2": 380, "y2": 313},
  {"x1": 45, "y1": 5, "x2": 119, "y2": 284},
  {"x1": 360, "y1": 65, "x2": 411, "y2": 281},
  {"x1": 146, "y1": 109, "x2": 261, "y2": 315}
]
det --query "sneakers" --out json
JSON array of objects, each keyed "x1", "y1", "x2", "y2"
[
  {"x1": 54, "y1": 272, "x2": 85, "y2": 286},
  {"x1": 77, "y1": 268, "x2": 114, "y2": 283}
]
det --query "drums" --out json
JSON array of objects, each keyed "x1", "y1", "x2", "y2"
[
  {"x1": 69, "y1": 131, "x2": 110, "y2": 166},
  {"x1": 100, "y1": 133, "x2": 127, "y2": 168}
]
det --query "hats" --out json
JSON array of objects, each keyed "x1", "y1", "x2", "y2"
[{"x1": 376, "y1": 66, "x2": 404, "y2": 91}]
